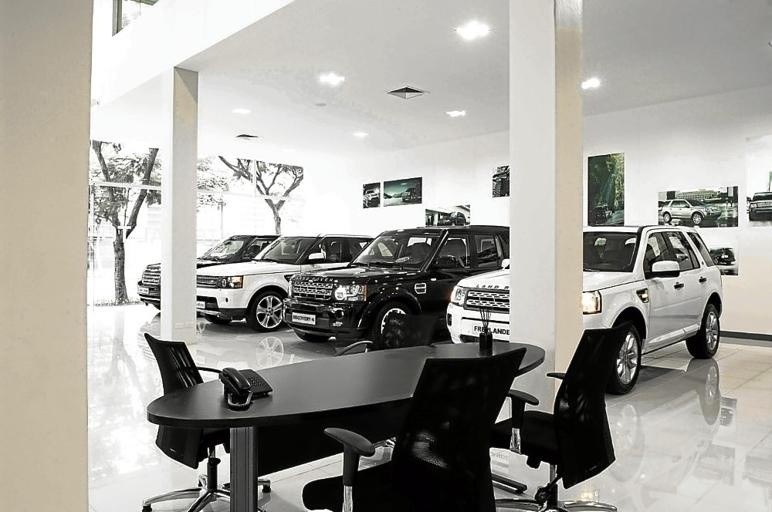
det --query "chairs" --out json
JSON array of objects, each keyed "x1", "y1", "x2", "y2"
[
  {"x1": 303, "y1": 347, "x2": 528, "y2": 511},
  {"x1": 403, "y1": 240, "x2": 465, "y2": 258},
  {"x1": 490, "y1": 321, "x2": 631, "y2": 510},
  {"x1": 144, "y1": 332, "x2": 271, "y2": 510},
  {"x1": 620, "y1": 243, "x2": 656, "y2": 271}
]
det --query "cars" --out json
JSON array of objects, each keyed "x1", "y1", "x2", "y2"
[{"x1": 746, "y1": 191, "x2": 771, "y2": 221}]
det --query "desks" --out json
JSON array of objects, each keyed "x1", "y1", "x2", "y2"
[{"x1": 147, "y1": 343, "x2": 546, "y2": 503}]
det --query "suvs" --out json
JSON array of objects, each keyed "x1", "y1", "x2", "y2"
[
  {"x1": 279, "y1": 225, "x2": 511, "y2": 351},
  {"x1": 590, "y1": 205, "x2": 613, "y2": 223},
  {"x1": 661, "y1": 198, "x2": 721, "y2": 224},
  {"x1": 196, "y1": 232, "x2": 379, "y2": 333},
  {"x1": 139, "y1": 234, "x2": 280, "y2": 311},
  {"x1": 363, "y1": 189, "x2": 378, "y2": 207},
  {"x1": 400, "y1": 188, "x2": 420, "y2": 202},
  {"x1": 445, "y1": 221, "x2": 723, "y2": 396}
]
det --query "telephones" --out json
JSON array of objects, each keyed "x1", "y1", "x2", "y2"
[{"x1": 219, "y1": 368, "x2": 273, "y2": 401}]
